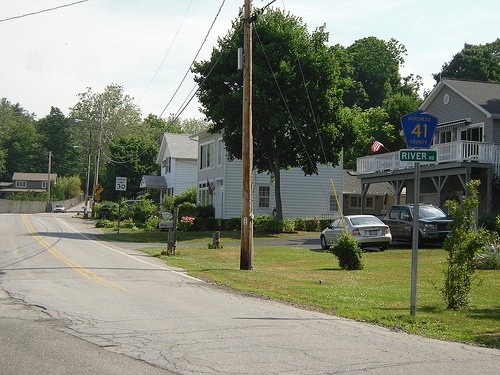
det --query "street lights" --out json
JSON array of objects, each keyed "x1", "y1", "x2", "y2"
[
  {"x1": 73, "y1": 146, "x2": 91, "y2": 208},
  {"x1": 76, "y1": 119, "x2": 102, "y2": 219}
]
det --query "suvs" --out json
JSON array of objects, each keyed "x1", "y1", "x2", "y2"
[{"x1": 383, "y1": 203, "x2": 456, "y2": 247}]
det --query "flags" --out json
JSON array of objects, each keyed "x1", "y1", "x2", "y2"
[{"x1": 370, "y1": 141, "x2": 383, "y2": 152}]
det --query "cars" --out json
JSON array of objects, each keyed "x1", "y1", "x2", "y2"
[
  {"x1": 53, "y1": 203, "x2": 67, "y2": 212},
  {"x1": 320, "y1": 214, "x2": 392, "y2": 250}
]
